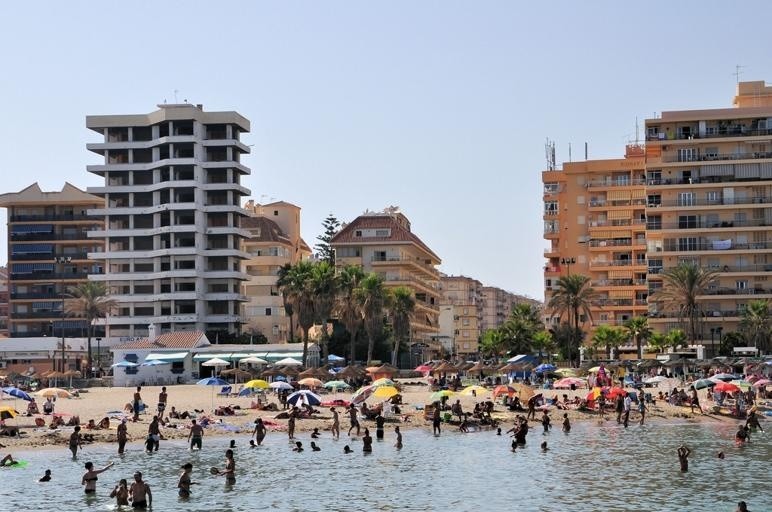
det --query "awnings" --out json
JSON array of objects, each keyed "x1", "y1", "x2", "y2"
[{"x1": 145, "y1": 351, "x2": 305, "y2": 362}]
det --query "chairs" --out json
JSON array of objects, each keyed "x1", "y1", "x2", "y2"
[
  {"x1": 217, "y1": 385, "x2": 232, "y2": 399},
  {"x1": 258, "y1": 394, "x2": 268, "y2": 406},
  {"x1": 278, "y1": 388, "x2": 289, "y2": 409}
]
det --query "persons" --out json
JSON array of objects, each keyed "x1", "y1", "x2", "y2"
[
  {"x1": 707, "y1": 384, "x2": 770, "y2": 446},
  {"x1": 431, "y1": 393, "x2": 579, "y2": 453},
  {"x1": 678, "y1": 446, "x2": 691, "y2": 471},
  {"x1": 734, "y1": 500, "x2": 751, "y2": 512},
  {"x1": 647, "y1": 366, "x2": 703, "y2": 414},
  {"x1": 281, "y1": 389, "x2": 402, "y2": 452},
  {"x1": 718, "y1": 451, "x2": 725, "y2": 459},
  {"x1": 28, "y1": 385, "x2": 267, "y2": 509},
  {"x1": 587, "y1": 365, "x2": 649, "y2": 427}
]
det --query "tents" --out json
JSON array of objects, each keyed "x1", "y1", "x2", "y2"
[{"x1": 320, "y1": 354, "x2": 346, "y2": 367}]
